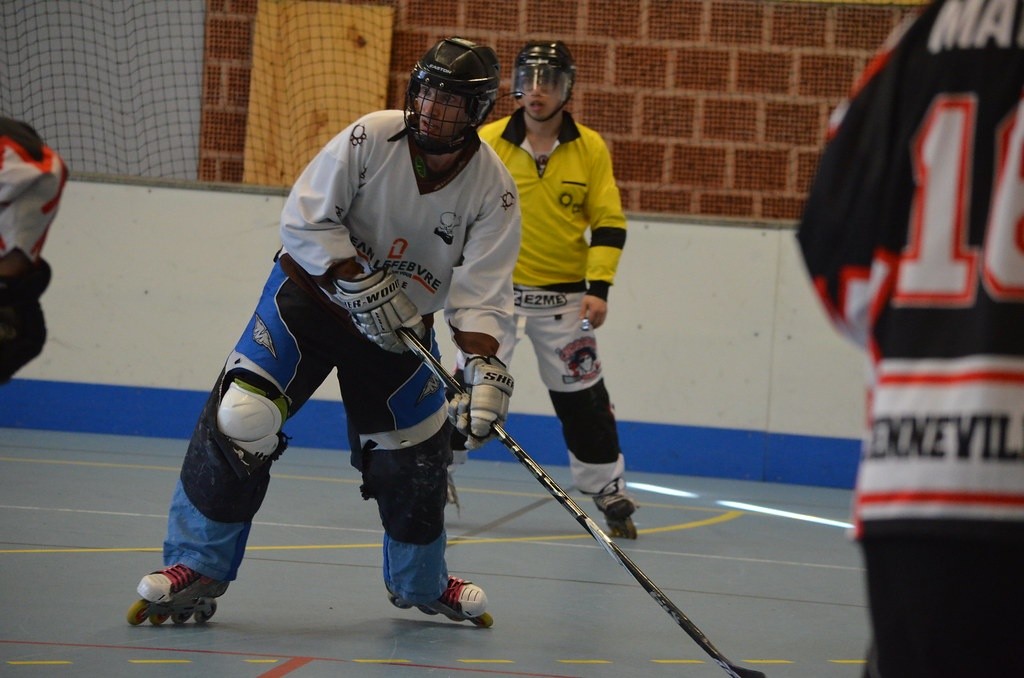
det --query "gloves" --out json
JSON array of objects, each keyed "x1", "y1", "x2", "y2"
[
  {"x1": 444, "y1": 354, "x2": 514, "y2": 451},
  {"x1": 333, "y1": 264, "x2": 426, "y2": 356}
]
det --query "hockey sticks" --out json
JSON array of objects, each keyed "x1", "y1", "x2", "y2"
[{"x1": 396, "y1": 323, "x2": 768, "y2": 678}]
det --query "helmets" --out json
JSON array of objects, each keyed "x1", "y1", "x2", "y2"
[
  {"x1": 402, "y1": 38, "x2": 502, "y2": 156},
  {"x1": 512, "y1": 40, "x2": 575, "y2": 102}
]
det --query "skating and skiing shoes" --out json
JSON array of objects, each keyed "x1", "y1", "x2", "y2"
[
  {"x1": 388, "y1": 574, "x2": 494, "y2": 628},
  {"x1": 592, "y1": 496, "x2": 638, "y2": 540},
  {"x1": 127, "y1": 563, "x2": 230, "y2": 627}
]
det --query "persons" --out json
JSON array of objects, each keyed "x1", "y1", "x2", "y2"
[
  {"x1": 127, "y1": 37, "x2": 519, "y2": 626},
  {"x1": 446, "y1": 40, "x2": 638, "y2": 539},
  {"x1": 0, "y1": 117, "x2": 69, "y2": 384},
  {"x1": 795, "y1": 0, "x2": 1024, "y2": 678}
]
